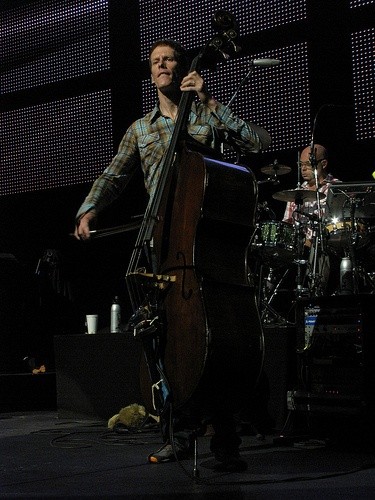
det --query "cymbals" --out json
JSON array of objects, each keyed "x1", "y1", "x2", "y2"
[
  {"x1": 272, "y1": 188, "x2": 327, "y2": 203},
  {"x1": 260, "y1": 162, "x2": 293, "y2": 177}
]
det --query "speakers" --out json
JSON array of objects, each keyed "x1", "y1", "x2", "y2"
[{"x1": 303, "y1": 293, "x2": 375, "y2": 398}]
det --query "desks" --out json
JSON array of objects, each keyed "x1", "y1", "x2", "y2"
[{"x1": 54, "y1": 332, "x2": 142, "y2": 419}]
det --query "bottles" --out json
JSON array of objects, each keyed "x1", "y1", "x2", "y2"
[
  {"x1": 110, "y1": 296, "x2": 121, "y2": 333},
  {"x1": 340, "y1": 258, "x2": 352, "y2": 295}
]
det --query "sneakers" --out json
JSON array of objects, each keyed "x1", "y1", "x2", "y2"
[
  {"x1": 148, "y1": 430, "x2": 196, "y2": 464},
  {"x1": 213, "y1": 446, "x2": 248, "y2": 472}
]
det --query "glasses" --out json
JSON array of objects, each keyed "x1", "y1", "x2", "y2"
[{"x1": 297, "y1": 158, "x2": 324, "y2": 170}]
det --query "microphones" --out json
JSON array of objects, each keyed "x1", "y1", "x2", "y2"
[{"x1": 250, "y1": 58, "x2": 281, "y2": 66}]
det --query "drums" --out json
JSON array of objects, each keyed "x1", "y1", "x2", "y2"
[
  {"x1": 322, "y1": 215, "x2": 371, "y2": 253},
  {"x1": 248, "y1": 218, "x2": 304, "y2": 268}
]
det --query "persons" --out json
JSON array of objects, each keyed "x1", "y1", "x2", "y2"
[
  {"x1": 75, "y1": 39, "x2": 265, "y2": 469},
  {"x1": 282, "y1": 144, "x2": 339, "y2": 247}
]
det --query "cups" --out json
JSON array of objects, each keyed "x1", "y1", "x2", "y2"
[{"x1": 86, "y1": 315, "x2": 98, "y2": 334}]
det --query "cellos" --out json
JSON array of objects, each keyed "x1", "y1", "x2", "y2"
[{"x1": 123, "y1": 7, "x2": 263, "y2": 472}]
